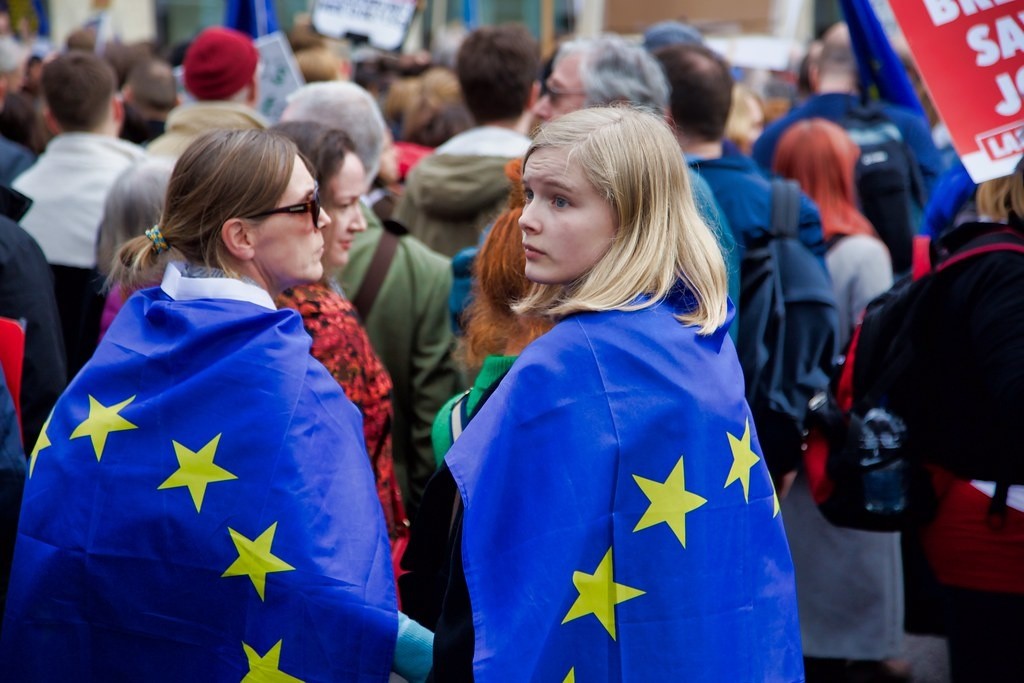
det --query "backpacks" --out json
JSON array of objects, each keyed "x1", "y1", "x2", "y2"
[
  {"x1": 732, "y1": 175, "x2": 840, "y2": 491},
  {"x1": 824, "y1": 100, "x2": 922, "y2": 275}
]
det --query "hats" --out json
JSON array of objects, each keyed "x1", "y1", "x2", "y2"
[
  {"x1": 184, "y1": 26, "x2": 257, "y2": 101},
  {"x1": 642, "y1": 20, "x2": 703, "y2": 57}
]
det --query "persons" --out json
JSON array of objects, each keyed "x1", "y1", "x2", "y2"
[
  {"x1": 1, "y1": 129, "x2": 399, "y2": 683},
  {"x1": 1, "y1": 0, "x2": 1024, "y2": 683},
  {"x1": 400, "y1": 104, "x2": 805, "y2": 683}
]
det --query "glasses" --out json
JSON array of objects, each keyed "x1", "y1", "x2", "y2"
[{"x1": 244, "y1": 181, "x2": 319, "y2": 229}]
